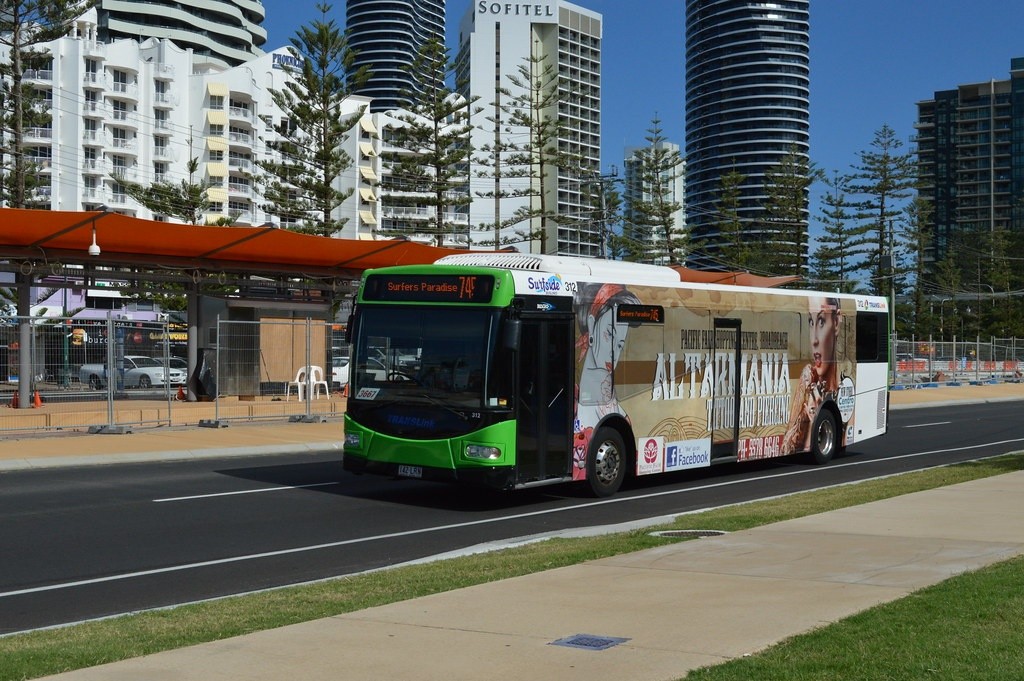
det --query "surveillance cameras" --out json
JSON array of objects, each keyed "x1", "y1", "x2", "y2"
[{"x1": 88, "y1": 245, "x2": 100, "y2": 257}]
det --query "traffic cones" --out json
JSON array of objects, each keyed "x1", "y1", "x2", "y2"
[
  {"x1": 344, "y1": 383, "x2": 349, "y2": 396},
  {"x1": 33, "y1": 389, "x2": 43, "y2": 407},
  {"x1": 12, "y1": 392, "x2": 19, "y2": 407},
  {"x1": 177, "y1": 385, "x2": 185, "y2": 398}
]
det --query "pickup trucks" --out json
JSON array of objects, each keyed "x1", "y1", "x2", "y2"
[{"x1": 79, "y1": 356, "x2": 183, "y2": 389}]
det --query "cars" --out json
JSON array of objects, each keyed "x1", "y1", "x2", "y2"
[{"x1": 153, "y1": 358, "x2": 189, "y2": 384}]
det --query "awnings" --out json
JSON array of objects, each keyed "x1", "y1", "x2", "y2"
[{"x1": 0, "y1": 203, "x2": 515, "y2": 411}]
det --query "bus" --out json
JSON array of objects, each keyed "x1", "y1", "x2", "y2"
[{"x1": 342, "y1": 252, "x2": 889, "y2": 498}]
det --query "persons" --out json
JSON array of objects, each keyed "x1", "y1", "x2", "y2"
[{"x1": 779, "y1": 297, "x2": 846, "y2": 454}]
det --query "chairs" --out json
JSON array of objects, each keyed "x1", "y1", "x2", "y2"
[
  {"x1": 310, "y1": 366, "x2": 329, "y2": 401},
  {"x1": 287, "y1": 366, "x2": 315, "y2": 402}
]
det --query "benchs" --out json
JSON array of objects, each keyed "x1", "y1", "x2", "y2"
[{"x1": 888, "y1": 378, "x2": 1023, "y2": 391}]
